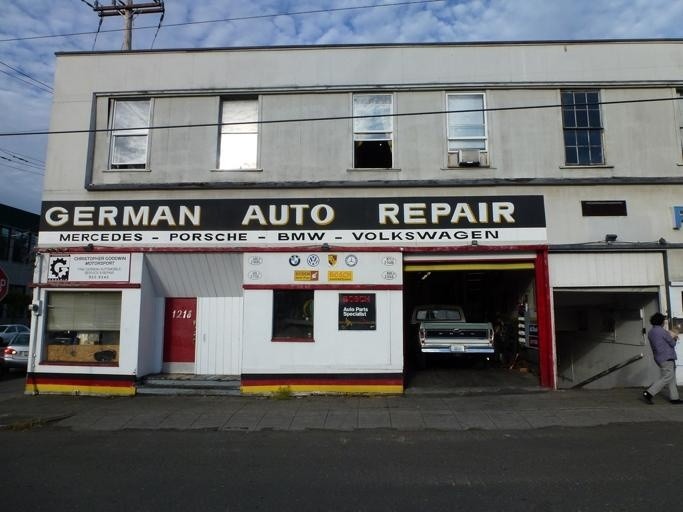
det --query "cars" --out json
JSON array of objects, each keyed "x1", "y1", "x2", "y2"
[{"x1": 0, "y1": 323, "x2": 71, "y2": 369}]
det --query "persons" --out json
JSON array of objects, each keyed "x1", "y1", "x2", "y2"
[{"x1": 641, "y1": 313, "x2": 681, "y2": 408}]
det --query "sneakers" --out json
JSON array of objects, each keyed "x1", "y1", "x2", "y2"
[
  {"x1": 641, "y1": 391, "x2": 653, "y2": 405},
  {"x1": 671, "y1": 399, "x2": 683, "y2": 404}
]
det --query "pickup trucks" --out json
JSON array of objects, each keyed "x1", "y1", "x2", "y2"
[{"x1": 405, "y1": 303, "x2": 495, "y2": 366}]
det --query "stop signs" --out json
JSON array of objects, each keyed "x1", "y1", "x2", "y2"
[{"x1": 0, "y1": 264, "x2": 9, "y2": 302}]
